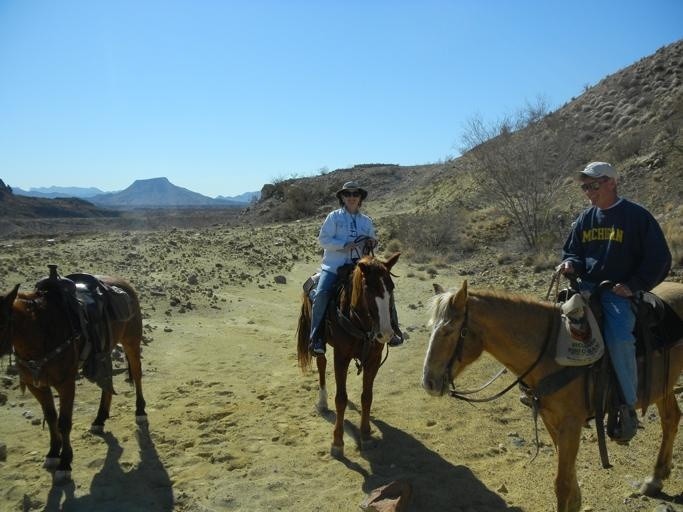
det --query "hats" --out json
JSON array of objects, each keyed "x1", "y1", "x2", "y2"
[
  {"x1": 578, "y1": 161, "x2": 619, "y2": 179},
  {"x1": 334, "y1": 181, "x2": 368, "y2": 199}
]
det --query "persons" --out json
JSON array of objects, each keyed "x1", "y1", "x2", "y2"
[
  {"x1": 307, "y1": 180, "x2": 403, "y2": 355},
  {"x1": 517, "y1": 159, "x2": 674, "y2": 445}
]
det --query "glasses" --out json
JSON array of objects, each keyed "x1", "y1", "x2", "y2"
[
  {"x1": 580, "y1": 181, "x2": 601, "y2": 192},
  {"x1": 340, "y1": 191, "x2": 361, "y2": 197}
]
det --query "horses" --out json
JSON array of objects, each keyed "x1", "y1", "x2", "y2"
[
  {"x1": 422, "y1": 280, "x2": 683, "y2": 512},
  {"x1": 295, "y1": 252, "x2": 404, "y2": 457},
  {"x1": 0, "y1": 275, "x2": 147, "y2": 486}
]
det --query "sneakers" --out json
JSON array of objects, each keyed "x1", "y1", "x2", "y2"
[
  {"x1": 517, "y1": 393, "x2": 541, "y2": 411},
  {"x1": 387, "y1": 333, "x2": 403, "y2": 348},
  {"x1": 611, "y1": 404, "x2": 639, "y2": 445},
  {"x1": 313, "y1": 340, "x2": 324, "y2": 354}
]
